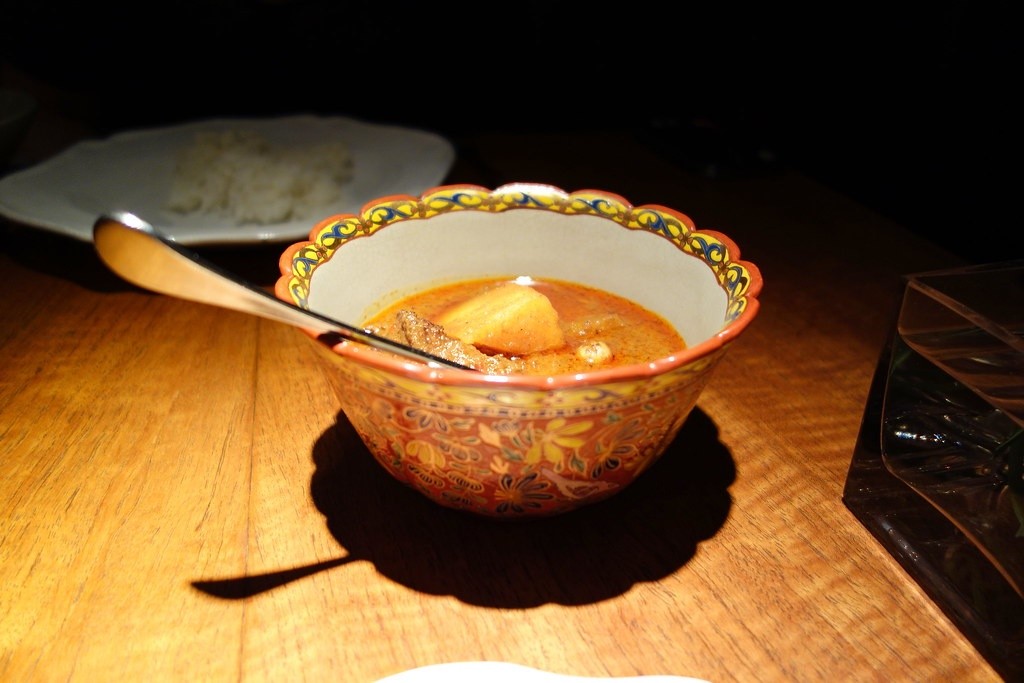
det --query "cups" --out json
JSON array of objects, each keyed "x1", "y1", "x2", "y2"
[{"x1": 844, "y1": 261, "x2": 1024, "y2": 683}]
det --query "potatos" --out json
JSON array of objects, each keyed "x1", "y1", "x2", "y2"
[{"x1": 438, "y1": 283, "x2": 565, "y2": 355}]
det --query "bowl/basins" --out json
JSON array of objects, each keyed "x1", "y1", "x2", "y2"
[{"x1": 273, "y1": 179, "x2": 764, "y2": 521}]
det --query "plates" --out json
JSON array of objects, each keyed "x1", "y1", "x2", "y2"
[{"x1": 0, "y1": 115, "x2": 456, "y2": 257}]
